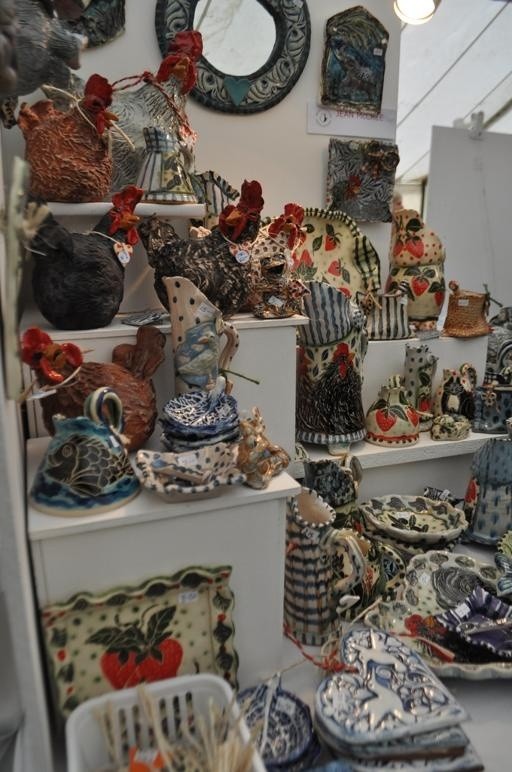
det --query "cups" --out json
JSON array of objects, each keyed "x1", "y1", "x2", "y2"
[{"x1": 282, "y1": 492, "x2": 362, "y2": 643}]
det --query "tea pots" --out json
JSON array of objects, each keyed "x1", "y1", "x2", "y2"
[
  {"x1": 29, "y1": 385, "x2": 143, "y2": 518},
  {"x1": 360, "y1": 375, "x2": 421, "y2": 449},
  {"x1": 133, "y1": 125, "x2": 199, "y2": 204}
]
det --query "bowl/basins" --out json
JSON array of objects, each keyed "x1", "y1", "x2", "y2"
[{"x1": 235, "y1": 689, "x2": 323, "y2": 771}]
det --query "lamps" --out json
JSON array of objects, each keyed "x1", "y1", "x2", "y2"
[{"x1": 393, "y1": 0, "x2": 442, "y2": 25}]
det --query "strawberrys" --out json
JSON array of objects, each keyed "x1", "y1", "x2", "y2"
[
  {"x1": 385, "y1": 215, "x2": 446, "y2": 308},
  {"x1": 293, "y1": 223, "x2": 352, "y2": 299},
  {"x1": 106, "y1": 605, "x2": 183, "y2": 682},
  {"x1": 85, "y1": 614, "x2": 146, "y2": 690},
  {"x1": 372, "y1": 391, "x2": 431, "y2": 431}
]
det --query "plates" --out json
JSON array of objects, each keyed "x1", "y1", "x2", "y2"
[
  {"x1": 323, "y1": 134, "x2": 400, "y2": 227},
  {"x1": 42, "y1": 566, "x2": 240, "y2": 719},
  {"x1": 259, "y1": 208, "x2": 382, "y2": 305},
  {"x1": 359, "y1": 493, "x2": 468, "y2": 544},
  {"x1": 137, "y1": 443, "x2": 246, "y2": 504},
  {"x1": 161, "y1": 390, "x2": 242, "y2": 453},
  {"x1": 433, "y1": 582, "x2": 512, "y2": 659},
  {"x1": 344, "y1": 548, "x2": 511, "y2": 678}
]
